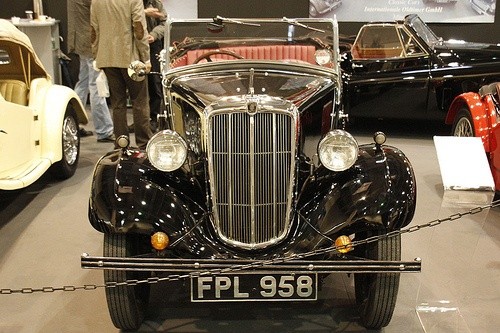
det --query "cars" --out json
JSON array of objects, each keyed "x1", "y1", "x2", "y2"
[
  {"x1": 0, "y1": 18, "x2": 90, "y2": 194},
  {"x1": 445, "y1": 81, "x2": 499, "y2": 208},
  {"x1": 80, "y1": 14, "x2": 424, "y2": 330},
  {"x1": 326, "y1": 13, "x2": 500, "y2": 121}
]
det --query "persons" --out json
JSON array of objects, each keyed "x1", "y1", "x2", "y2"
[
  {"x1": 128, "y1": 0, "x2": 167, "y2": 134},
  {"x1": 66, "y1": 0, "x2": 118, "y2": 142},
  {"x1": 90, "y1": 0, "x2": 153, "y2": 151}
]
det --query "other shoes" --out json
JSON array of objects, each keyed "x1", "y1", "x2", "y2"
[
  {"x1": 79, "y1": 127, "x2": 93, "y2": 136},
  {"x1": 97, "y1": 134, "x2": 116, "y2": 142}
]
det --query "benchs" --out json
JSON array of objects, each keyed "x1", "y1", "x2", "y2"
[{"x1": 170, "y1": 46, "x2": 316, "y2": 68}]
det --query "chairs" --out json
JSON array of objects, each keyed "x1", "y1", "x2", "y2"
[{"x1": 0, "y1": 79, "x2": 27, "y2": 106}]
